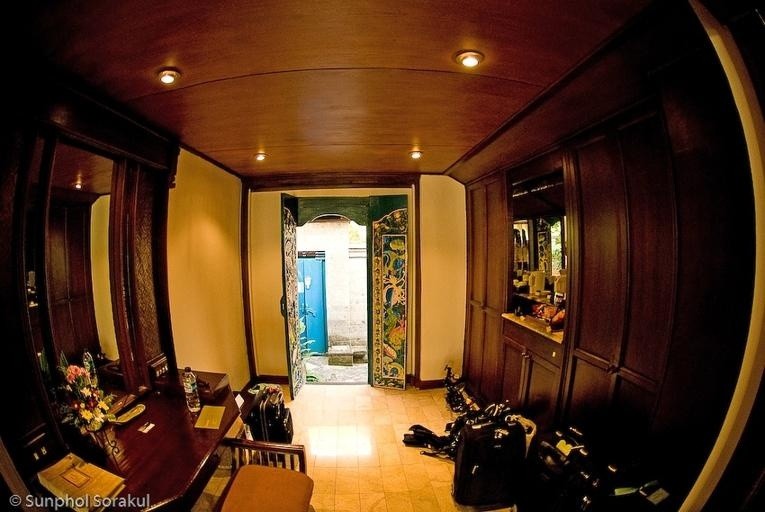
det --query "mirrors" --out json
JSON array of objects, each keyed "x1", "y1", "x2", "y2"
[{"x1": 23, "y1": 124, "x2": 140, "y2": 428}]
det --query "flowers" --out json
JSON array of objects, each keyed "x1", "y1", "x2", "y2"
[{"x1": 48, "y1": 350, "x2": 118, "y2": 430}]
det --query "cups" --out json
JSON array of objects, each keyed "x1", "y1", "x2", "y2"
[{"x1": 529, "y1": 272, "x2": 546, "y2": 295}]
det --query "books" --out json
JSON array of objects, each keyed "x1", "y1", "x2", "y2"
[{"x1": 37, "y1": 452, "x2": 127, "y2": 512}]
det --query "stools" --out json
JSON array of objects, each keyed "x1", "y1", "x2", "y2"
[{"x1": 208, "y1": 438, "x2": 314, "y2": 511}]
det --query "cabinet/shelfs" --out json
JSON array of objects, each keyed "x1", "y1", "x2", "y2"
[{"x1": 460, "y1": 2, "x2": 764, "y2": 441}]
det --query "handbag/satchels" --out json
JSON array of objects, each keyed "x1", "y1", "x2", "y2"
[{"x1": 446, "y1": 404, "x2": 537, "y2": 458}]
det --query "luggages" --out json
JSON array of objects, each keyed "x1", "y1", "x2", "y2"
[
  {"x1": 248, "y1": 385, "x2": 294, "y2": 462},
  {"x1": 450, "y1": 418, "x2": 528, "y2": 508},
  {"x1": 517, "y1": 424, "x2": 675, "y2": 512}
]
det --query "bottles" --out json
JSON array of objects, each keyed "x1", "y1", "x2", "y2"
[
  {"x1": 182, "y1": 367, "x2": 200, "y2": 413},
  {"x1": 554, "y1": 268, "x2": 567, "y2": 293},
  {"x1": 82, "y1": 347, "x2": 99, "y2": 391},
  {"x1": 511, "y1": 226, "x2": 521, "y2": 269},
  {"x1": 521, "y1": 228, "x2": 531, "y2": 269}
]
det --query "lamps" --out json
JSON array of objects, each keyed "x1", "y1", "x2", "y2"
[{"x1": 25, "y1": 270, "x2": 39, "y2": 309}]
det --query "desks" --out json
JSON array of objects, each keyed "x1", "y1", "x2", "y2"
[{"x1": 0, "y1": 366, "x2": 240, "y2": 512}]
